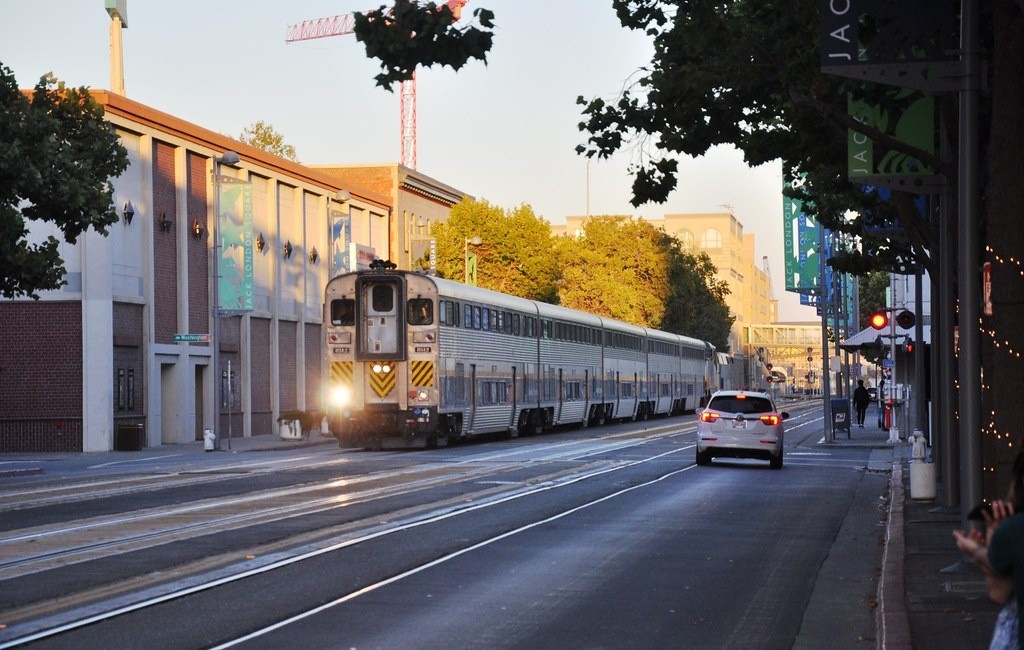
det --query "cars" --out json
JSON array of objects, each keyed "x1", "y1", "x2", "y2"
[{"x1": 695, "y1": 389, "x2": 791, "y2": 469}]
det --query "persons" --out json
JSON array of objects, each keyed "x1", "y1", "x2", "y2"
[
  {"x1": 876, "y1": 380, "x2": 885, "y2": 428},
  {"x1": 340, "y1": 302, "x2": 354, "y2": 322},
  {"x1": 951, "y1": 446, "x2": 1024, "y2": 650},
  {"x1": 852, "y1": 380, "x2": 869, "y2": 427}
]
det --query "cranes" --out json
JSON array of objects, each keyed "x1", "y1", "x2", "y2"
[{"x1": 284, "y1": 1, "x2": 468, "y2": 173}]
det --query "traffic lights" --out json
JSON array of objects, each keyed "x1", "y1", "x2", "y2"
[
  {"x1": 871, "y1": 313, "x2": 888, "y2": 330},
  {"x1": 896, "y1": 311, "x2": 916, "y2": 329},
  {"x1": 767, "y1": 364, "x2": 773, "y2": 370},
  {"x1": 902, "y1": 336, "x2": 913, "y2": 353},
  {"x1": 767, "y1": 377, "x2": 773, "y2": 382}
]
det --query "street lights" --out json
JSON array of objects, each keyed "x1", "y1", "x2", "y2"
[{"x1": 465, "y1": 233, "x2": 484, "y2": 284}]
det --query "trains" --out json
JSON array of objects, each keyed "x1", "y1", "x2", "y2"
[{"x1": 321, "y1": 258, "x2": 770, "y2": 452}]
